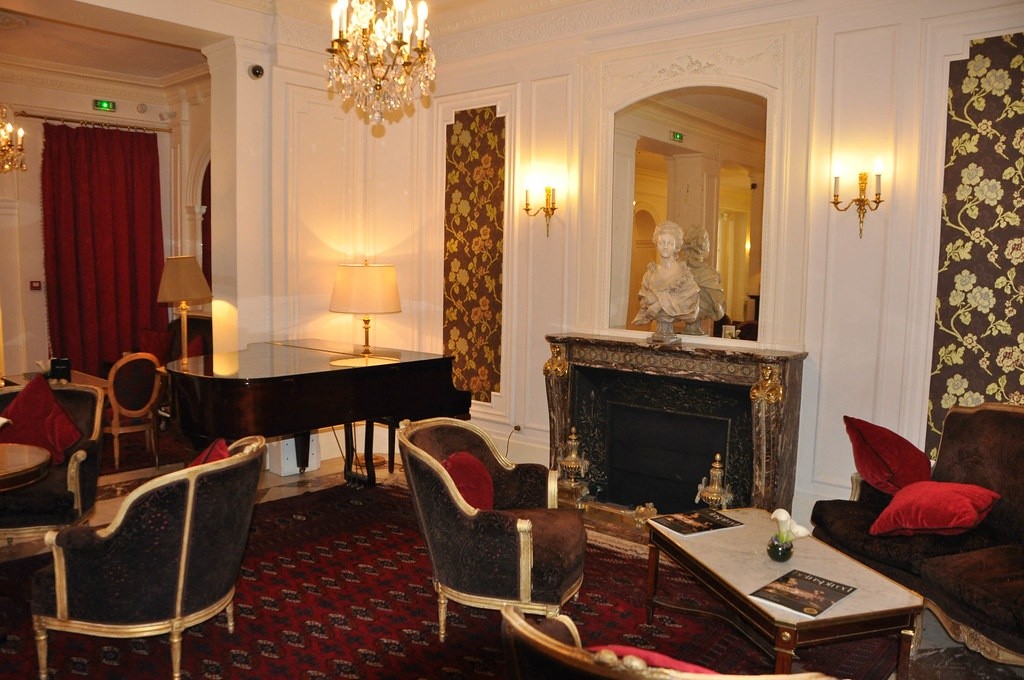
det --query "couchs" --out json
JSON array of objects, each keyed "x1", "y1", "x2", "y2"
[{"x1": 809, "y1": 401, "x2": 1024, "y2": 669}]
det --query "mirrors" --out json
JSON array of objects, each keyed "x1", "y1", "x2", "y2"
[{"x1": 607, "y1": 84, "x2": 768, "y2": 340}]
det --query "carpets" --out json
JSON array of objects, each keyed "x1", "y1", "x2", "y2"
[{"x1": 0, "y1": 481, "x2": 899, "y2": 680}]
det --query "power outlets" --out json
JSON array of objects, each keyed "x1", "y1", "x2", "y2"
[{"x1": 511, "y1": 423, "x2": 523, "y2": 433}]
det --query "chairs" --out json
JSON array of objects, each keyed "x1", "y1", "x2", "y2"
[
  {"x1": 0, "y1": 382, "x2": 108, "y2": 519},
  {"x1": 101, "y1": 353, "x2": 167, "y2": 468},
  {"x1": 17, "y1": 433, "x2": 271, "y2": 680},
  {"x1": 394, "y1": 416, "x2": 588, "y2": 640},
  {"x1": 500, "y1": 601, "x2": 841, "y2": 680}
]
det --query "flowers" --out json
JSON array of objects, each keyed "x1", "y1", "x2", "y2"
[{"x1": 768, "y1": 507, "x2": 811, "y2": 543}]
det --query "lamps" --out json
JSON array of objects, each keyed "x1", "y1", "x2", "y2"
[
  {"x1": 556, "y1": 424, "x2": 591, "y2": 510},
  {"x1": 523, "y1": 185, "x2": 558, "y2": 238},
  {"x1": 829, "y1": 171, "x2": 885, "y2": 238},
  {"x1": 0, "y1": 105, "x2": 28, "y2": 174},
  {"x1": 327, "y1": 0, "x2": 437, "y2": 138},
  {"x1": 694, "y1": 453, "x2": 734, "y2": 510},
  {"x1": 155, "y1": 254, "x2": 214, "y2": 357},
  {"x1": 327, "y1": 259, "x2": 404, "y2": 357}
]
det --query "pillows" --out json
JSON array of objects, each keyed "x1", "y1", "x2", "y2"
[
  {"x1": 187, "y1": 435, "x2": 229, "y2": 467},
  {"x1": 841, "y1": 413, "x2": 932, "y2": 496},
  {"x1": 869, "y1": 479, "x2": 1002, "y2": 537},
  {"x1": 0, "y1": 374, "x2": 83, "y2": 466},
  {"x1": 440, "y1": 450, "x2": 493, "y2": 511}
]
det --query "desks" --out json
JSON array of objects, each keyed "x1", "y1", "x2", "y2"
[
  {"x1": 0, "y1": 369, "x2": 109, "y2": 403},
  {"x1": 0, "y1": 443, "x2": 52, "y2": 493}
]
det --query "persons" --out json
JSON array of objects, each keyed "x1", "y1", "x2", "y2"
[
  {"x1": 683, "y1": 224, "x2": 727, "y2": 320},
  {"x1": 631, "y1": 221, "x2": 700, "y2": 324}
]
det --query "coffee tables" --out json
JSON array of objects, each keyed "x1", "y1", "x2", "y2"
[{"x1": 640, "y1": 505, "x2": 925, "y2": 680}]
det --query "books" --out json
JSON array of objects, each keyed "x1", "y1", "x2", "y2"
[
  {"x1": 650, "y1": 509, "x2": 744, "y2": 537},
  {"x1": 748, "y1": 570, "x2": 857, "y2": 619}
]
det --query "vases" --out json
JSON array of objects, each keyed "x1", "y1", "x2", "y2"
[{"x1": 765, "y1": 536, "x2": 794, "y2": 562}]
